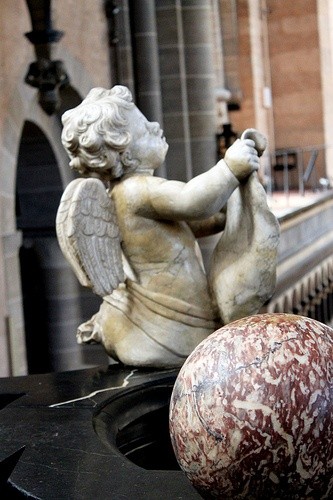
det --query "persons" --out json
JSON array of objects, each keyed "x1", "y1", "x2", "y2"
[{"x1": 61, "y1": 84, "x2": 261, "y2": 369}]
